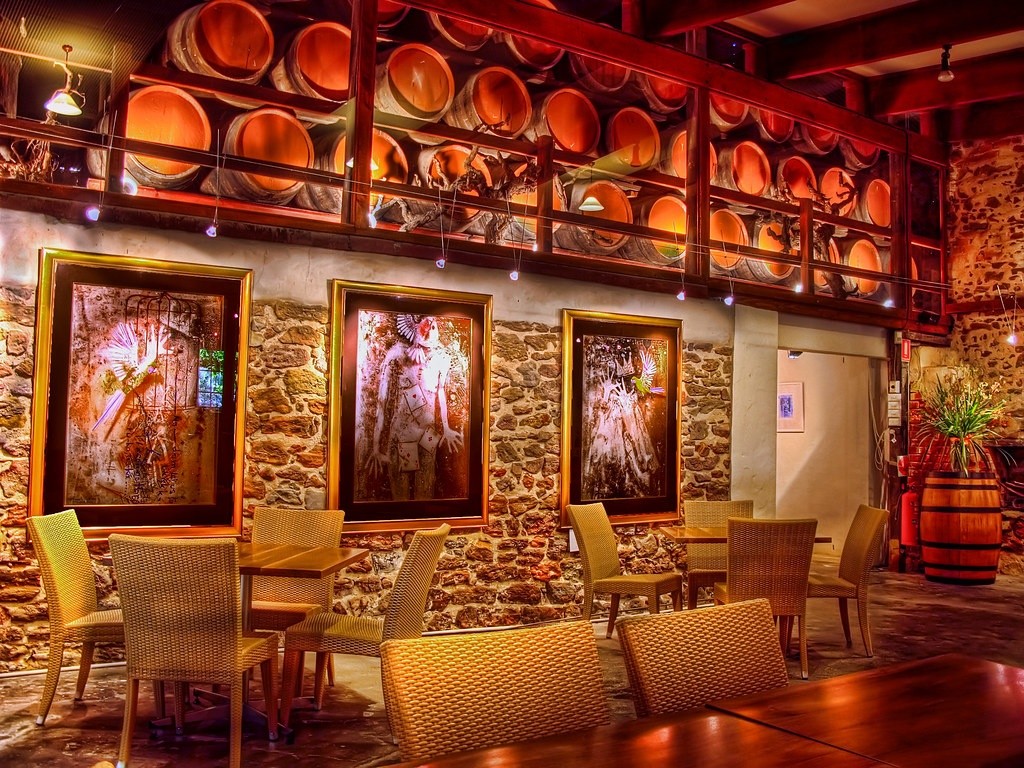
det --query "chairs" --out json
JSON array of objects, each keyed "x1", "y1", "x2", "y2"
[
  {"x1": 789, "y1": 505, "x2": 888, "y2": 678},
  {"x1": 249, "y1": 508, "x2": 346, "y2": 698},
  {"x1": 614, "y1": 597, "x2": 789, "y2": 720},
  {"x1": 378, "y1": 620, "x2": 611, "y2": 761},
  {"x1": 564, "y1": 502, "x2": 684, "y2": 638},
  {"x1": 107, "y1": 534, "x2": 276, "y2": 768},
  {"x1": 281, "y1": 524, "x2": 454, "y2": 742},
  {"x1": 25, "y1": 509, "x2": 166, "y2": 726},
  {"x1": 683, "y1": 499, "x2": 754, "y2": 610},
  {"x1": 713, "y1": 517, "x2": 818, "y2": 678}
]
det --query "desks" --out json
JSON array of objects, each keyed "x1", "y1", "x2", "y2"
[
  {"x1": 387, "y1": 655, "x2": 1024, "y2": 768},
  {"x1": 657, "y1": 524, "x2": 833, "y2": 610}
]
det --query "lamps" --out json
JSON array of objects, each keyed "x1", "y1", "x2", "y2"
[
  {"x1": 720, "y1": 230, "x2": 741, "y2": 305},
  {"x1": 206, "y1": 129, "x2": 226, "y2": 237},
  {"x1": 674, "y1": 214, "x2": 689, "y2": 301},
  {"x1": 506, "y1": 187, "x2": 528, "y2": 279},
  {"x1": 45, "y1": 45, "x2": 83, "y2": 116},
  {"x1": 937, "y1": 43, "x2": 954, "y2": 82},
  {"x1": 579, "y1": 161, "x2": 605, "y2": 210},
  {"x1": 436, "y1": 178, "x2": 456, "y2": 268},
  {"x1": 788, "y1": 350, "x2": 802, "y2": 359},
  {"x1": 87, "y1": 108, "x2": 116, "y2": 220},
  {"x1": 996, "y1": 285, "x2": 1016, "y2": 344}
]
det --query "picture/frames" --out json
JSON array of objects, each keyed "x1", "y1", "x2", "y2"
[
  {"x1": 777, "y1": 382, "x2": 804, "y2": 432},
  {"x1": 558, "y1": 309, "x2": 682, "y2": 530},
  {"x1": 26, "y1": 248, "x2": 251, "y2": 544},
  {"x1": 327, "y1": 279, "x2": 499, "y2": 532}
]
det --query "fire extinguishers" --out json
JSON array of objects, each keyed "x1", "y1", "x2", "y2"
[{"x1": 901, "y1": 481, "x2": 919, "y2": 547}]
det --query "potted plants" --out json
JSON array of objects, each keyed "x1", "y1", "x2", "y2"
[{"x1": 916, "y1": 362, "x2": 1014, "y2": 583}]
